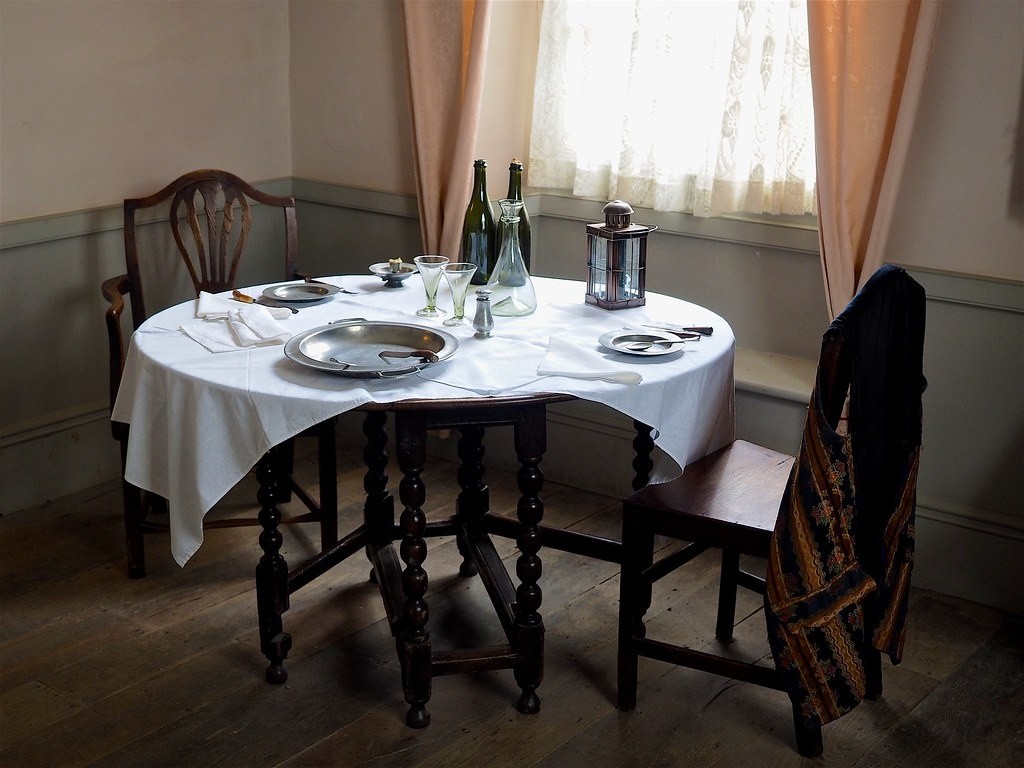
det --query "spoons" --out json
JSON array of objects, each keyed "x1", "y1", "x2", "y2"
[{"x1": 625, "y1": 335, "x2": 700, "y2": 350}]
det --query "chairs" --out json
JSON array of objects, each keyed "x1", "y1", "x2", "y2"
[
  {"x1": 102, "y1": 170, "x2": 339, "y2": 582},
  {"x1": 617, "y1": 264, "x2": 927, "y2": 759}
]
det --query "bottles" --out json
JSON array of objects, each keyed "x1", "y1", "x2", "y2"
[
  {"x1": 472, "y1": 288, "x2": 495, "y2": 337},
  {"x1": 494, "y1": 156, "x2": 533, "y2": 288},
  {"x1": 454, "y1": 158, "x2": 499, "y2": 287},
  {"x1": 484, "y1": 198, "x2": 538, "y2": 317}
]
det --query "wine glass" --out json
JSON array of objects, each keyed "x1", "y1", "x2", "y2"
[
  {"x1": 439, "y1": 261, "x2": 478, "y2": 327},
  {"x1": 413, "y1": 255, "x2": 450, "y2": 320}
]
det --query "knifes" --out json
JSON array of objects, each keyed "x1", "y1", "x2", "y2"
[
  {"x1": 304, "y1": 276, "x2": 360, "y2": 294},
  {"x1": 378, "y1": 349, "x2": 439, "y2": 364},
  {"x1": 641, "y1": 325, "x2": 714, "y2": 335}
]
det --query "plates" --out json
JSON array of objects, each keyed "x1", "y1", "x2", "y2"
[
  {"x1": 262, "y1": 283, "x2": 340, "y2": 303},
  {"x1": 598, "y1": 330, "x2": 687, "y2": 356},
  {"x1": 284, "y1": 317, "x2": 461, "y2": 380}
]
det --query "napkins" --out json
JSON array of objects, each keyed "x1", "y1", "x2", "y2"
[
  {"x1": 538, "y1": 335, "x2": 644, "y2": 385},
  {"x1": 198, "y1": 291, "x2": 292, "y2": 323}
]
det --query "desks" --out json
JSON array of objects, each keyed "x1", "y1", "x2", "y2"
[{"x1": 108, "y1": 272, "x2": 735, "y2": 727}]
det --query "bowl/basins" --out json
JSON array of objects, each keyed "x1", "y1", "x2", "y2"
[{"x1": 368, "y1": 262, "x2": 419, "y2": 289}]
